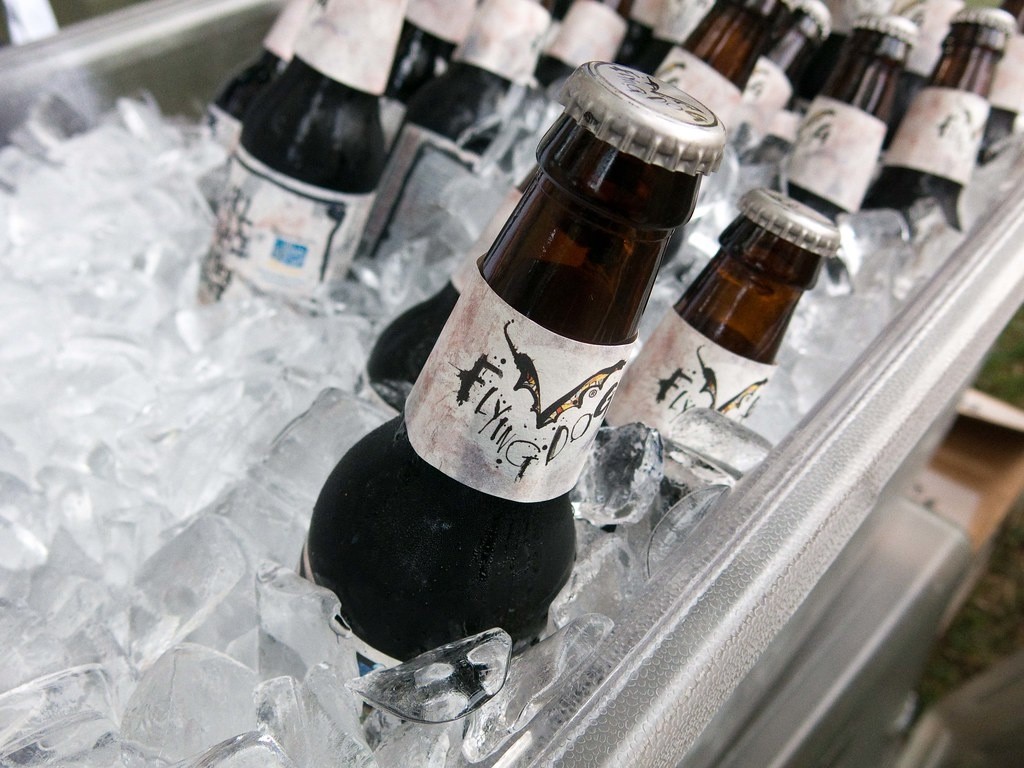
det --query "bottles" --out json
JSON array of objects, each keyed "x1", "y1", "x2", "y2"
[{"x1": 199, "y1": 0, "x2": 1024, "y2": 705}]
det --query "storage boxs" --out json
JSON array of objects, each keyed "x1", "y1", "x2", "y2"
[{"x1": 907, "y1": 385, "x2": 1023, "y2": 557}]
